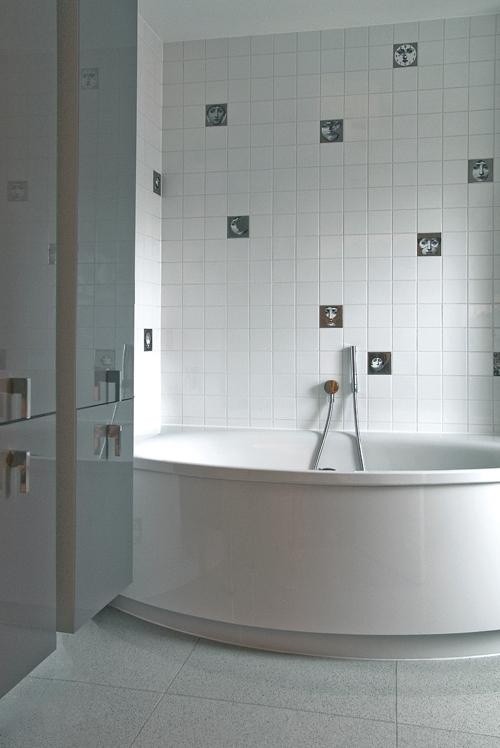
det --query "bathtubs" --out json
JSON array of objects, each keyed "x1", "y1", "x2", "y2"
[{"x1": 106, "y1": 426, "x2": 499, "y2": 661}]
